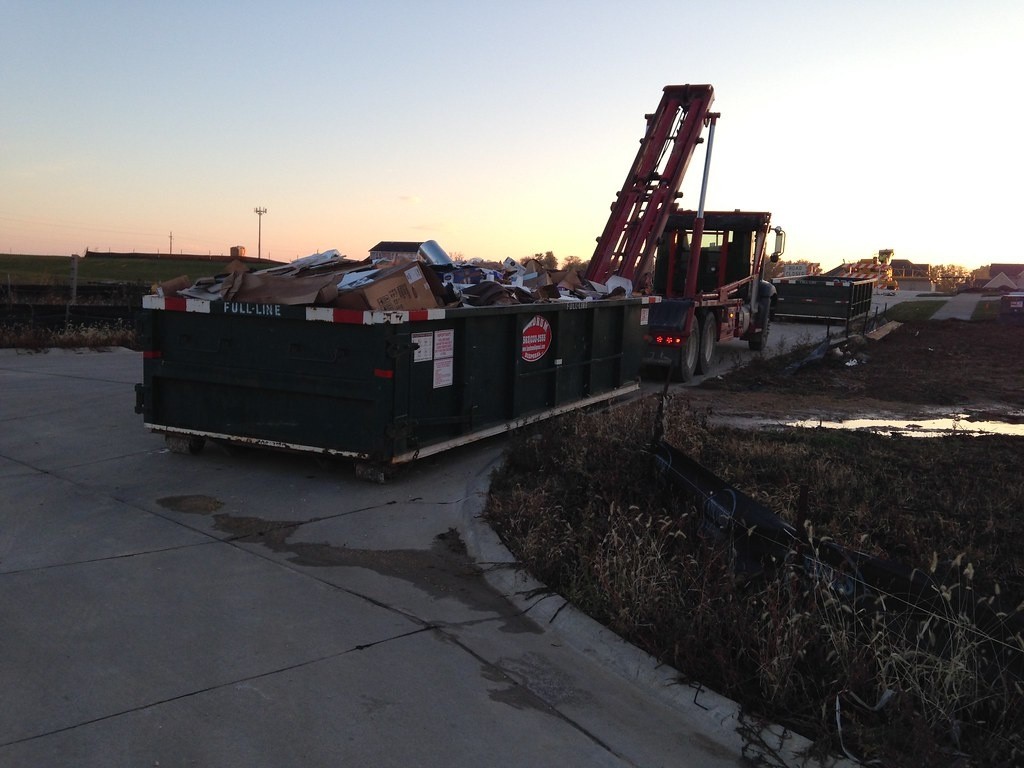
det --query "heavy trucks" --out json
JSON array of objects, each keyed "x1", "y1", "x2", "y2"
[{"x1": 134, "y1": 81, "x2": 785, "y2": 485}]
